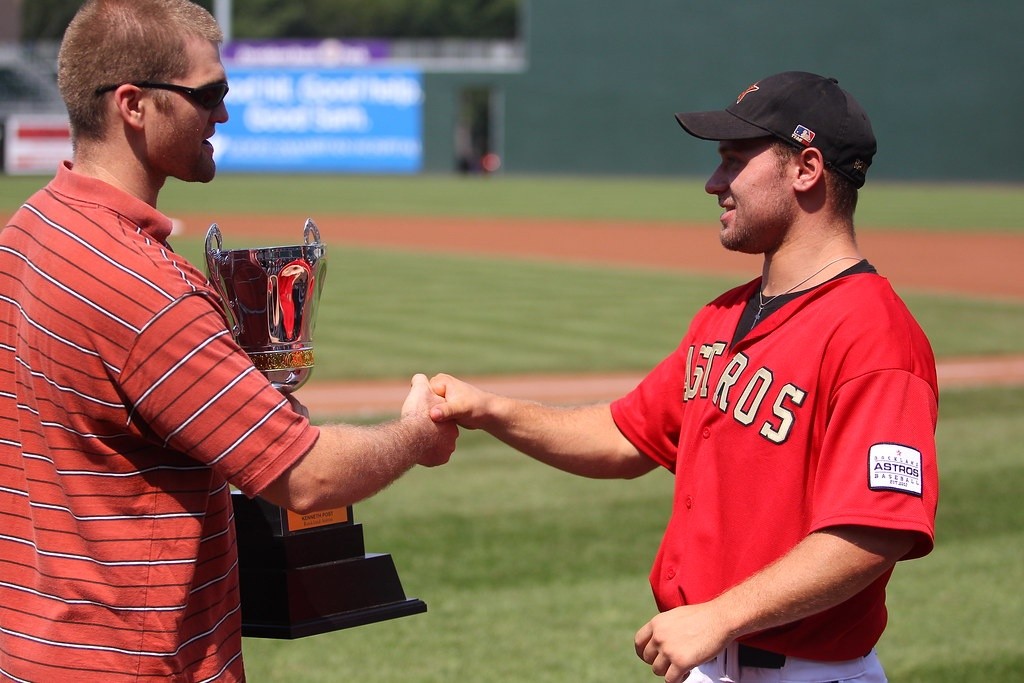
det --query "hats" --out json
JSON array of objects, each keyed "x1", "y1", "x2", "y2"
[{"x1": 676, "y1": 71, "x2": 878, "y2": 189}]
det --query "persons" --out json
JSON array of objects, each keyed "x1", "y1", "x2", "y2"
[
  {"x1": 0, "y1": 0, "x2": 459, "y2": 681},
  {"x1": 427, "y1": 71, "x2": 938, "y2": 682}
]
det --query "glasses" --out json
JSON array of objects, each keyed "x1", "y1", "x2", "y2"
[{"x1": 95, "y1": 78, "x2": 230, "y2": 110}]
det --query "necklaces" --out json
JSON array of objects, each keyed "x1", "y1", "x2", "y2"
[{"x1": 752, "y1": 257, "x2": 860, "y2": 327}]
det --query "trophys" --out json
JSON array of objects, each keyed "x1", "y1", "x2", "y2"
[{"x1": 204, "y1": 220, "x2": 426, "y2": 639}]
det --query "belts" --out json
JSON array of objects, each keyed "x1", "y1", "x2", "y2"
[{"x1": 715, "y1": 643, "x2": 786, "y2": 669}]
